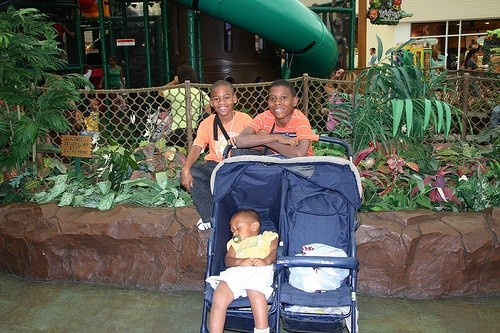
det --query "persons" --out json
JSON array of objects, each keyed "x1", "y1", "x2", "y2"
[
  {"x1": 354, "y1": 48, "x2": 414, "y2": 76},
  {"x1": 159, "y1": 65, "x2": 211, "y2": 147},
  {"x1": 79, "y1": 56, "x2": 125, "y2": 135},
  {"x1": 447, "y1": 39, "x2": 483, "y2": 70},
  {"x1": 237, "y1": 80, "x2": 311, "y2": 157},
  {"x1": 325, "y1": 69, "x2": 358, "y2": 139},
  {"x1": 180, "y1": 80, "x2": 252, "y2": 231},
  {"x1": 208, "y1": 209, "x2": 278, "y2": 333}
]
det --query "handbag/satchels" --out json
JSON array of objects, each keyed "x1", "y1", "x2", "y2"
[{"x1": 289, "y1": 243, "x2": 349, "y2": 294}]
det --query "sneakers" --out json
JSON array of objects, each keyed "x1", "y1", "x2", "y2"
[{"x1": 196, "y1": 218, "x2": 211, "y2": 231}]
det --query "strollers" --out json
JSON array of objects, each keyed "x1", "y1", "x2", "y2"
[
  {"x1": 199, "y1": 131, "x2": 277, "y2": 333},
  {"x1": 277, "y1": 134, "x2": 363, "y2": 333}
]
